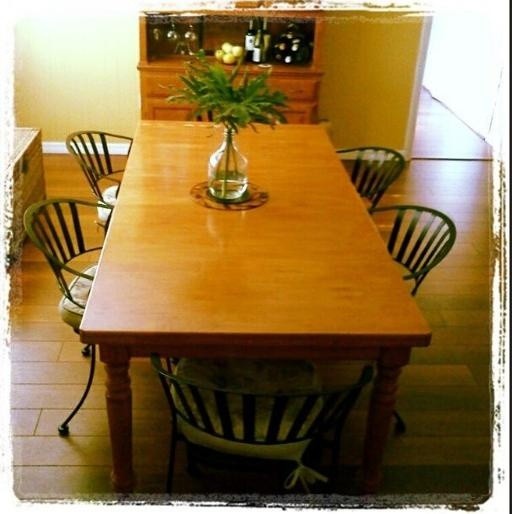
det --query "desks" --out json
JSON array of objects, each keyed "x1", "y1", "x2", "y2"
[{"x1": 80, "y1": 120, "x2": 433, "y2": 493}]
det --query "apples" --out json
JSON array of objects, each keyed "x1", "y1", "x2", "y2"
[
  {"x1": 221, "y1": 42, "x2": 232, "y2": 53},
  {"x1": 223, "y1": 53, "x2": 235, "y2": 64},
  {"x1": 214, "y1": 49, "x2": 224, "y2": 61},
  {"x1": 231, "y1": 46, "x2": 243, "y2": 56}
]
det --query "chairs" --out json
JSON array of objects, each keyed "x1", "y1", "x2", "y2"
[
  {"x1": 147, "y1": 352, "x2": 373, "y2": 494},
  {"x1": 65, "y1": 129, "x2": 133, "y2": 226},
  {"x1": 367, "y1": 205, "x2": 457, "y2": 433},
  {"x1": 24, "y1": 198, "x2": 115, "y2": 436},
  {"x1": 335, "y1": 146, "x2": 406, "y2": 209}
]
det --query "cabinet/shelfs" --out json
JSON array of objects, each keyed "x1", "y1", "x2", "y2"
[
  {"x1": 137, "y1": 57, "x2": 324, "y2": 123},
  {"x1": 6, "y1": 127, "x2": 47, "y2": 272}
]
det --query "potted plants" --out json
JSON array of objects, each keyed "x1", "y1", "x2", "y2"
[{"x1": 160, "y1": 49, "x2": 290, "y2": 200}]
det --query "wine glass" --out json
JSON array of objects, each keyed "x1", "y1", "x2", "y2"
[{"x1": 151, "y1": 19, "x2": 198, "y2": 43}]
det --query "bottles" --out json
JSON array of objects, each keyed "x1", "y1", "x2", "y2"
[{"x1": 245, "y1": 16, "x2": 269, "y2": 62}]
